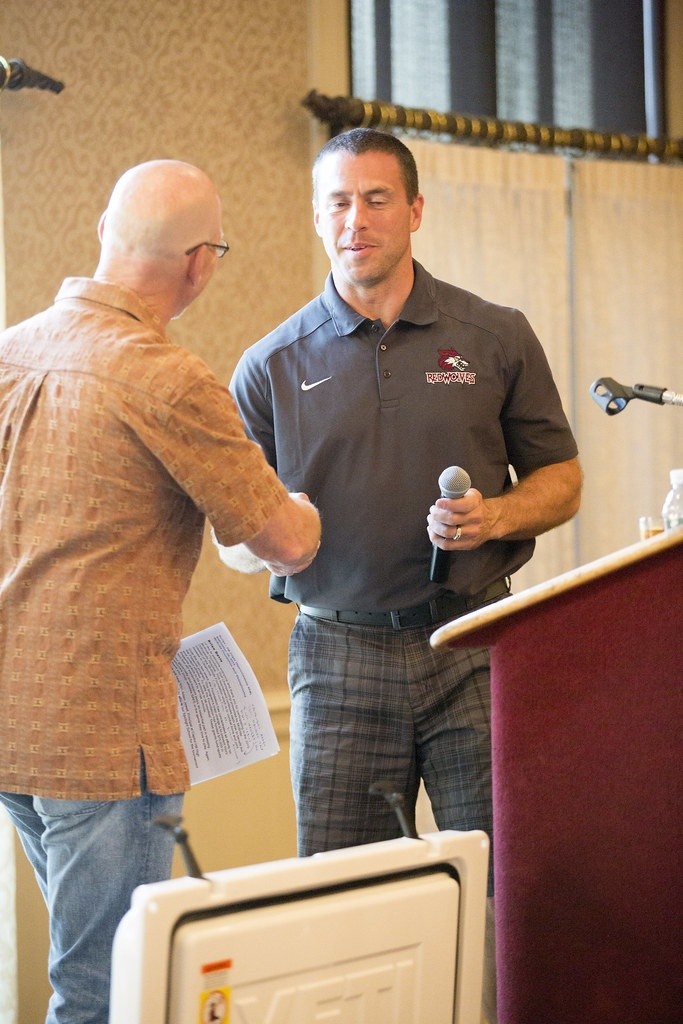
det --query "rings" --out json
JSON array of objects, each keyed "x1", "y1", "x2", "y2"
[{"x1": 453, "y1": 525, "x2": 462, "y2": 540}]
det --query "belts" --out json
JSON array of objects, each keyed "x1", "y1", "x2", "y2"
[{"x1": 300, "y1": 579, "x2": 509, "y2": 629}]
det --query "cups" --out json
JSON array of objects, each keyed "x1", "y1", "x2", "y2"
[{"x1": 638, "y1": 516, "x2": 664, "y2": 540}]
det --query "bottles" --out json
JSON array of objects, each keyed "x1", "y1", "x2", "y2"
[{"x1": 662, "y1": 468, "x2": 683, "y2": 531}]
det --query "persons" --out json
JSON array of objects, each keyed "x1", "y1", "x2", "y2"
[
  {"x1": 212, "y1": 129, "x2": 583, "y2": 1024},
  {"x1": 1, "y1": 161, "x2": 321, "y2": 1024}
]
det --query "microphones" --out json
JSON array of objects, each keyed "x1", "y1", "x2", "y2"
[{"x1": 430, "y1": 466, "x2": 471, "y2": 582}]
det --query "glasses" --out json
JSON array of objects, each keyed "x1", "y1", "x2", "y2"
[{"x1": 187, "y1": 239, "x2": 231, "y2": 259}]
